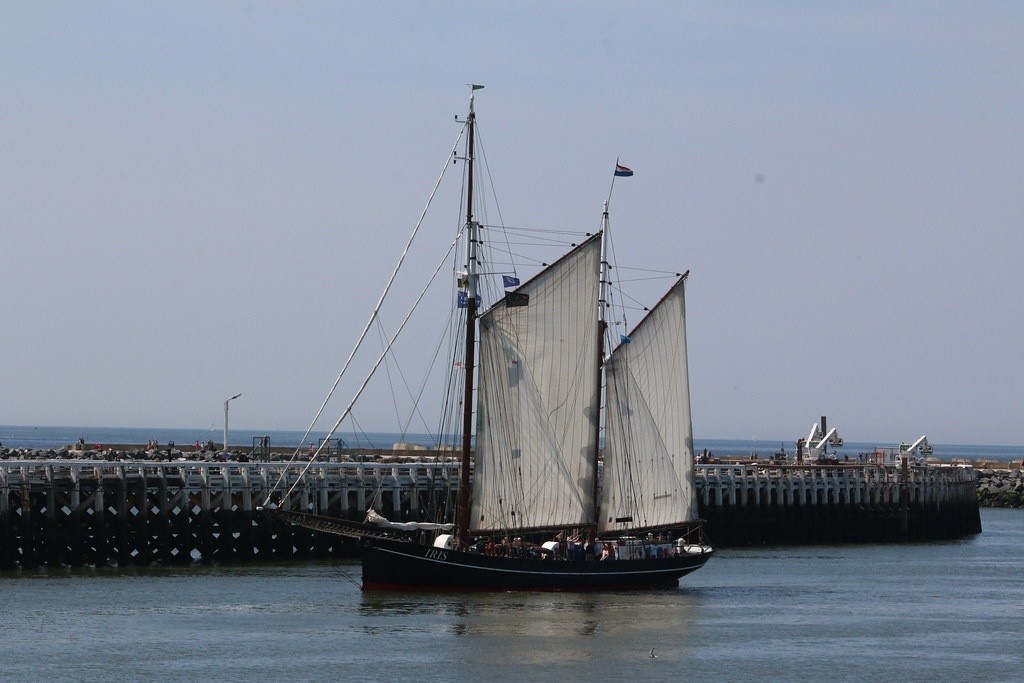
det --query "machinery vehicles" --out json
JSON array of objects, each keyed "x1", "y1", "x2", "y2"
[
  {"x1": 792, "y1": 422, "x2": 843, "y2": 465},
  {"x1": 895, "y1": 435, "x2": 935, "y2": 476}
]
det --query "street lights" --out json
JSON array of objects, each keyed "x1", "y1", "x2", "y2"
[{"x1": 223, "y1": 392, "x2": 242, "y2": 462}]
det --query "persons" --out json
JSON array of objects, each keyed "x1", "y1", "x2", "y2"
[
  {"x1": 703, "y1": 447, "x2": 712, "y2": 458},
  {"x1": 750, "y1": 452, "x2": 758, "y2": 460},
  {"x1": 797, "y1": 439, "x2": 803, "y2": 466},
  {"x1": 827, "y1": 450, "x2": 863, "y2": 462},
  {"x1": 0, "y1": 438, "x2": 216, "y2": 453},
  {"x1": 450, "y1": 528, "x2": 685, "y2": 561}
]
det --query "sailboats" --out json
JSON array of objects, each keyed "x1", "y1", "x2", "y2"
[{"x1": 256, "y1": 78, "x2": 717, "y2": 592}]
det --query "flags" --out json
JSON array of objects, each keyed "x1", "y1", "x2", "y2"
[
  {"x1": 613, "y1": 164, "x2": 633, "y2": 177},
  {"x1": 504, "y1": 290, "x2": 530, "y2": 307},
  {"x1": 620, "y1": 334, "x2": 632, "y2": 345},
  {"x1": 457, "y1": 278, "x2": 471, "y2": 289},
  {"x1": 502, "y1": 275, "x2": 520, "y2": 288},
  {"x1": 457, "y1": 289, "x2": 481, "y2": 308}
]
